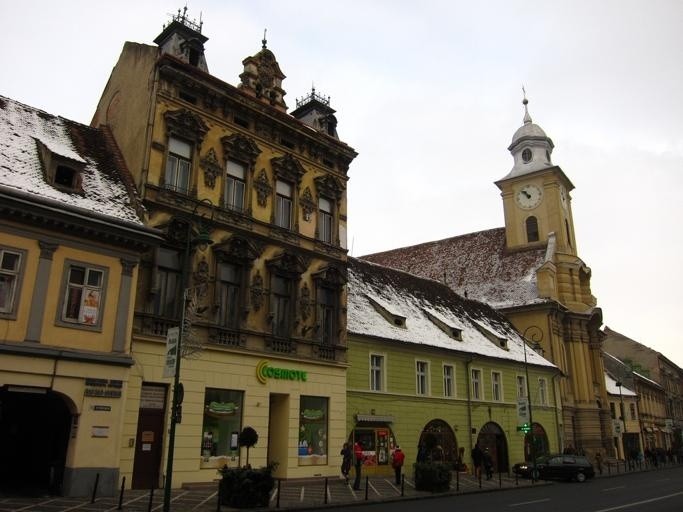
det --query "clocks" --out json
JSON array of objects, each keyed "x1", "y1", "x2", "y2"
[
  {"x1": 560, "y1": 185, "x2": 569, "y2": 210},
  {"x1": 514, "y1": 183, "x2": 543, "y2": 210}
]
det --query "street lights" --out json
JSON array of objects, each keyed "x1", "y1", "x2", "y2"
[
  {"x1": 521, "y1": 324, "x2": 545, "y2": 483},
  {"x1": 616, "y1": 360, "x2": 634, "y2": 471},
  {"x1": 160, "y1": 198, "x2": 216, "y2": 512}
]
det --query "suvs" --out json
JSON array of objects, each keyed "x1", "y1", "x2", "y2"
[{"x1": 512, "y1": 454, "x2": 594, "y2": 482}]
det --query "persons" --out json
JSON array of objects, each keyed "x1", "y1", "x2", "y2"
[
  {"x1": 471, "y1": 443, "x2": 482, "y2": 478},
  {"x1": 630, "y1": 443, "x2": 683, "y2": 473},
  {"x1": 390, "y1": 444, "x2": 405, "y2": 485},
  {"x1": 352, "y1": 445, "x2": 363, "y2": 490},
  {"x1": 594, "y1": 448, "x2": 604, "y2": 474},
  {"x1": 481, "y1": 448, "x2": 493, "y2": 482},
  {"x1": 565, "y1": 443, "x2": 586, "y2": 456},
  {"x1": 339, "y1": 442, "x2": 352, "y2": 485}
]
